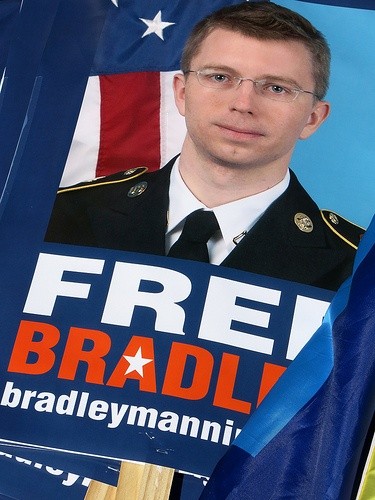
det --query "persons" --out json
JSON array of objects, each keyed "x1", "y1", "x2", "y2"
[{"x1": 37, "y1": 2, "x2": 374, "y2": 295}]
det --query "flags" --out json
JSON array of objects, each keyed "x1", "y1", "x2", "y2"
[
  {"x1": 58, "y1": 70, "x2": 197, "y2": 188},
  {"x1": 77, "y1": 0, "x2": 239, "y2": 74}
]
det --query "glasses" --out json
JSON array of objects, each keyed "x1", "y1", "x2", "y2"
[{"x1": 185, "y1": 63, "x2": 319, "y2": 108}]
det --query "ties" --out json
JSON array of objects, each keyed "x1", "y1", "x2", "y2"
[{"x1": 162, "y1": 208, "x2": 222, "y2": 265}]
